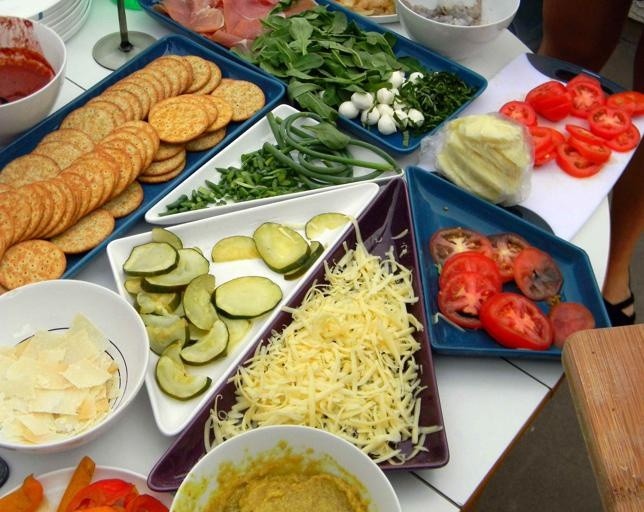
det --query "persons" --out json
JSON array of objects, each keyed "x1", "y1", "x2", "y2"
[
  {"x1": 511, "y1": 1, "x2": 633, "y2": 74},
  {"x1": 601, "y1": 28, "x2": 644, "y2": 325}
]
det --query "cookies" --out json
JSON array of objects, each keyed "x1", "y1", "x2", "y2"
[{"x1": 0, "y1": 55, "x2": 265, "y2": 296}]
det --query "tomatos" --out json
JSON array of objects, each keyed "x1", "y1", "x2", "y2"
[
  {"x1": 430, "y1": 228, "x2": 595, "y2": 350},
  {"x1": 499, "y1": 73, "x2": 644, "y2": 178}
]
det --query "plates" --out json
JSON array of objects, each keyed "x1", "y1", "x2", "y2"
[
  {"x1": 145, "y1": 175, "x2": 450, "y2": 494},
  {"x1": 137, "y1": 1, "x2": 489, "y2": 154},
  {"x1": 0, "y1": 463, "x2": 174, "y2": 512},
  {"x1": 106, "y1": 182, "x2": 381, "y2": 436},
  {"x1": 144, "y1": 103, "x2": 405, "y2": 224},
  {"x1": 1, "y1": 0, "x2": 95, "y2": 41},
  {"x1": 404, "y1": 163, "x2": 612, "y2": 357},
  {"x1": 0, "y1": 33, "x2": 285, "y2": 286}
]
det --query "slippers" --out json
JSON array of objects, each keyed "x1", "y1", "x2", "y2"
[{"x1": 604, "y1": 264, "x2": 635, "y2": 326}]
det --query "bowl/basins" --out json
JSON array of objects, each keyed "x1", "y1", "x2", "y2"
[
  {"x1": 1, "y1": 277, "x2": 152, "y2": 452},
  {"x1": 393, "y1": 1, "x2": 524, "y2": 65},
  {"x1": 0, "y1": 15, "x2": 68, "y2": 144},
  {"x1": 166, "y1": 425, "x2": 404, "y2": 512}
]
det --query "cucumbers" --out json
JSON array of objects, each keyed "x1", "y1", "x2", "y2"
[{"x1": 123, "y1": 214, "x2": 351, "y2": 401}]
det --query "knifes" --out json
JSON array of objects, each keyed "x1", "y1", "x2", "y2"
[{"x1": 526, "y1": 51, "x2": 628, "y2": 98}]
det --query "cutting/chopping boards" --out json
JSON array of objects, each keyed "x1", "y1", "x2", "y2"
[{"x1": 416, "y1": 51, "x2": 643, "y2": 243}]
